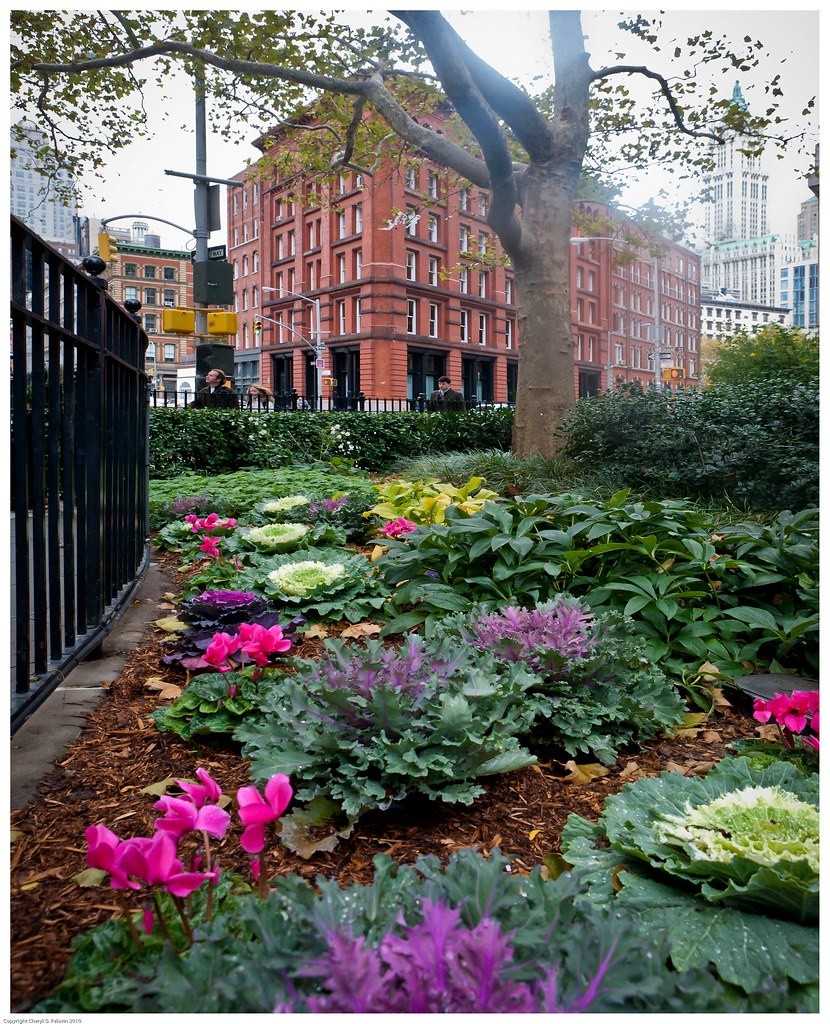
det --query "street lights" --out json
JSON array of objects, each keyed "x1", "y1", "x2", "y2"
[
  {"x1": 261, "y1": 286, "x2": 330, "y2": 414},
  {"x1": 569, "y1": 236, "x2": 663, "y2": 393},
  {"x1": 605, "y1": 323, "x2": 651, "y2": 394}
]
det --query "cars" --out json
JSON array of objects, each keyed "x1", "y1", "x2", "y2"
[{"x1": 470, "y1": 402, "x2": 514, "y2": 416}]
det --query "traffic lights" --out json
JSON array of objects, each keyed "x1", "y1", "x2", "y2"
[
  {"x1": 670, "y1": 368, "x2": 685, "y2": 380},
  {"x1": 322, "y1": 378, "x2": 332, "y2": 386},
  {"x1": 97, "y1": 231, "x2": 119, "y2": 263},
  {"x1": 254, "y1": 321, "x2": 261, "y2": 335}
]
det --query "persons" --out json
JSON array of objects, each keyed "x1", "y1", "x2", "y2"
[
  {"x1": 184, "y1": 369, "x2": 356, "y2": 411},
  {"x1": 427, "y1": 375, "x2": 466, "y2": 412}
]
